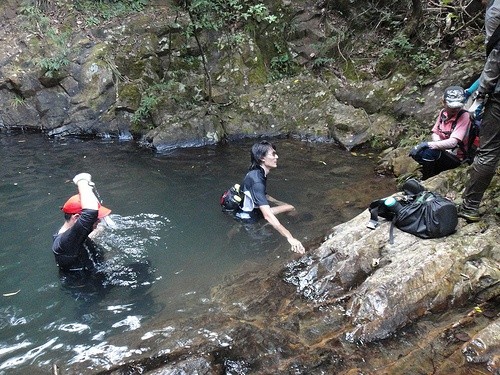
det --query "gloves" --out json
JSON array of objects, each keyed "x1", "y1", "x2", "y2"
[{"x1": 468, "y1": 98, "x2": 486, "y2": 121}]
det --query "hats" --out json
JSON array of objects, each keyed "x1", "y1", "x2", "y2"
[
  {"x1": 443, "y1": 86, "x2": 466, "y2": 108},
  {"x1": 60, "y1": 193, "x2": 111, "y2": 218}
]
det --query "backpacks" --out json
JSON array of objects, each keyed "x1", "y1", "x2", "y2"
[
  {"x1": 220, "y1": 174, "x2": 262, "y2": 217},
  {"x1": 408, "y1": 143, "x2": 462, "y2": 180},
  {"x1": 438, "y1": 110, "x2": 479, "y2": 166},
  {"x1": 369, "y1": 179, "x2": 459, "y2": 244}
]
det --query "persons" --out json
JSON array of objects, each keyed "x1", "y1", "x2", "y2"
[
  {"x1": 51, "y1": 173, "x2": 166, "y2": 315},
  {"x1": 409, "y1": 0, "x2": 500, "y2": 222},
  {"x1": 227, "y1": 140, "x2": 305, "y2": 254}
]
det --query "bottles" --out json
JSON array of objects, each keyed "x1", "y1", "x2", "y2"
[
  {"x1": 385, "y1": 198, "x2": 403, "y2": 213},
  {"x1": 222, "y1": 195, "x2": 242, "y2": 216}
]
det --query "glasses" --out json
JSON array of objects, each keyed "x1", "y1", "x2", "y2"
[{"x1": 264, "y1": 151, "x2": 279, "y2": 157}]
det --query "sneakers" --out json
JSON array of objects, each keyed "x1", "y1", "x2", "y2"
[{"x1": 454, "y1": 199, "x2": 480, "y2": 221}]
